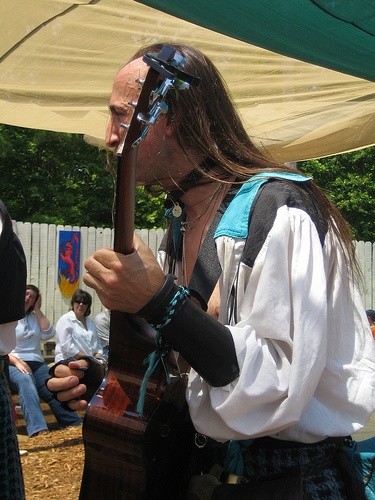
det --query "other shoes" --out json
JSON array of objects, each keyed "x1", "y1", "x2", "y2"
[{"x1": 36, "y1": 429, "x2": 48, "y2": 436}]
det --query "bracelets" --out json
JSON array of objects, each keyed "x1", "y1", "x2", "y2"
[{"x1": 137, "y1": 284, "x2": 191, "y2": 422}]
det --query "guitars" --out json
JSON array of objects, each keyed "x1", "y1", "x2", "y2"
[{"x1": 76, "y1": 41, "x2": 223, "y2": 500}]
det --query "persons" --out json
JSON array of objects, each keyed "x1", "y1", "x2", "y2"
[
  {"x1": 365, "y1": 309, "x2": 375, "y2": 340},
  {"x1": 0, "y1": 199, "x2": 28, "y2": 500},
  {"x1": 54, "y1": 288, "x2": 104, "y2": 362},
  {"x1": 83, "y1": 42, "x2": 375, "y2": 500},
  {"x1": 8, "y1": 285, "x2": 84, "y2": 438},
  {"x1": 91, "y1": 308, "x2": 111, "y2": 362}
]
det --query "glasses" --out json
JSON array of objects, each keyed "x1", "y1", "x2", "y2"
[{"x1": 74, "y1": 297, "x2": 89, "y2": 305}]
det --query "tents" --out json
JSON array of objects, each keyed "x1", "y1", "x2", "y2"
[{"x1": 0, "y1": 0, "x2": 375, "y2": 183}]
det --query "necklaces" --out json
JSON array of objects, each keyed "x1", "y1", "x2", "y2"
[
  {"x1": 163, "y1": 153, "x2": 218, "y2": 218},
  {"x1": 179, "y1": 178, "x2": 224, "y2": 233}
]
